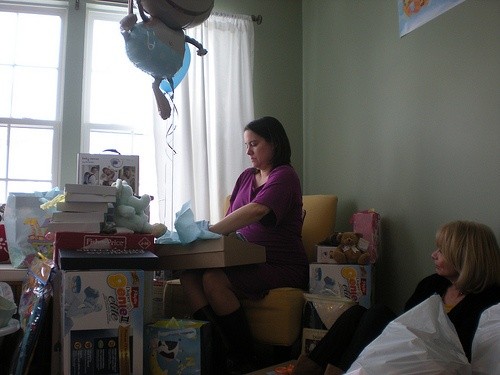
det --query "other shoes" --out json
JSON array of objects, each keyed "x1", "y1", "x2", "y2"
[{"x1": 274, "y1": 364, "x2": 297, "y2": 375}]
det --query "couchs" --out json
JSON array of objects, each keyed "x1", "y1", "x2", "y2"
[{"x1": 238, "y1": 194, "x2": 339, "y2": 347}]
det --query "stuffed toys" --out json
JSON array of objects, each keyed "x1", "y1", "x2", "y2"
[
  {"x1": 111, "y1": 178, "x2": 167, "y2": 239},
  {"x1": 333, "y1": 231, "x2": 370, "y2": 265}
]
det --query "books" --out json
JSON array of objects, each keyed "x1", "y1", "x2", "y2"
[
  {"x1": 65, "y1": 194, "x2": 117, "y2": 202},
  {"x1": 47, "y1": 202, "x2": 117, "y2": 233},
  {"x1": 64, "y1": 184, "x2": 117, "y2": 195}
]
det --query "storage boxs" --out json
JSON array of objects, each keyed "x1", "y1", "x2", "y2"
[
  {"x1": 154, "y1": 233, "x2": 266, "y2": 273},
  {"x1": 53, "y1": 211, "x2": 105, "y2": 223},
  {"x1": 301, "y1": 328, "x2": 330, "y2": 357},
  {"x1": 55, "y1": 202, "x2": 109, "y2": 214},
  {"x1": 53, "y1": 231, "x2": 154, "y2": 271},
  {"x1": 65, "y1": 194, "x2": 116, "y2": 203},
  {"x1": 303, "y1": 292, "x2": 359, "y2": 331},
  {"x1": 57, "y1": 248, "x2": 159, "y2": 271},
  {"x1": 314, "y1": 241, "x2": 346, "y2": 264},
  {"x1": 58, "y1": 269, "x2": 144, "y2": 375},
  {"x1": 47, "y1": 221, "x2": 103, "y2": 234},
  {"x1": 309, "y1": 263, "x2": 375, "y2": 304},
  {"x1": 64, "y1": 183, "x2": 116, "y2": 196},
  {"x1": 76, "y1": 152, "x2": 140, "y2": 209},
  {"x1": 142, "y1": 319, "x2": 212, "y2": 375}
]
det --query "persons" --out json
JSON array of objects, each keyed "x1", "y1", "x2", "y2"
[
  {"x1": 293, "y1": 220, "x2": 500, "y2": 375},
  {"x1": 101, "y1": 167, "x2": 116, "y2": 186},
  {"x1": 180, "y1": 116, "x2": 310, "y2": 375},
  {"x1": 83, "y1": 167, "x2": 98, "y2": 185}
]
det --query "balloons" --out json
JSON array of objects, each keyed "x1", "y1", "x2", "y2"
[
  {"x1": 158, "y1": 42, "x2": 191, "y2": 92},
  {"x1": 120, "y1": 0, "x2": 214, "y2": 120}
]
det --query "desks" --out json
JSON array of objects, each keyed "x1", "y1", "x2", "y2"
[{"x1": 0, "y1": 263, "x2": 31, "y2": 282}]
